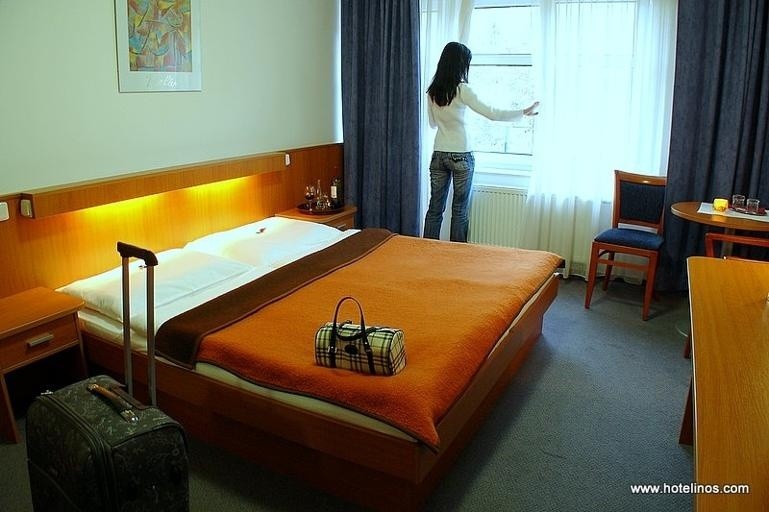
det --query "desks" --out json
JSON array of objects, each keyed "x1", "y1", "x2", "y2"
[
  {"x1": 670, "y1": 201, "x2": 769, "y2": 509},
  {"x1": 0, "y1": 284, "x2": 85, "y2": 444},
  {"x1": 274, "y1": 204, "x2": 358, "y2": 230},
  {"x1": 585, "y1": 168, "x2": 668, "y2": 321}
]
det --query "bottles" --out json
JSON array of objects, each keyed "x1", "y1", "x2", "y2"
[{"x1": 329, "y1": 166, "x2": 344, "y2": 207}]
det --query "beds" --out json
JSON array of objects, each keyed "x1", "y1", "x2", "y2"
[{"x1": 57, "y1": 217, "x2": 562, "y2": 511}]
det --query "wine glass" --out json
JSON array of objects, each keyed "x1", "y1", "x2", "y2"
[{"x1": 304, "y1": 185, "x2": 317, "y2": 212}]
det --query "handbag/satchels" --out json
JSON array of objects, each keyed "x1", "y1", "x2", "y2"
[{"x1": 312, "y1": 294, "x2": 408, "y2": 376}]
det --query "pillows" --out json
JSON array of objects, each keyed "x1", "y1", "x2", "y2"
[{"x1": 53, "y1": 218, "x2": 344, "y2": 324}]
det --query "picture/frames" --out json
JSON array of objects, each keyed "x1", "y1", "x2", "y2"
[{"x1": 113, "y1": 0, "x2": 203, "y2": 93}]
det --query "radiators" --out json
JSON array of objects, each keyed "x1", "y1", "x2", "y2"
[{"x1": 468, "y1": 186, "x2": 575, "y2": 282}]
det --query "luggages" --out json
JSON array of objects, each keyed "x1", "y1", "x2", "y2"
[{"x1": 24, "y1": 377, "x2": 190, "y2": 511}]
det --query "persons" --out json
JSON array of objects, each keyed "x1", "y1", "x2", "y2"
[{"x1": 424, "y1": 42, "x2": 539, "y2": 243}]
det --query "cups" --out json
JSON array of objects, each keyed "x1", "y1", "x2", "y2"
[
  {"x1": 747, "y1": 199, "x2": 760, "y2": 213},
  {"x1": 732, "y1": 194, "x2": 745, "y2": 208}
]
